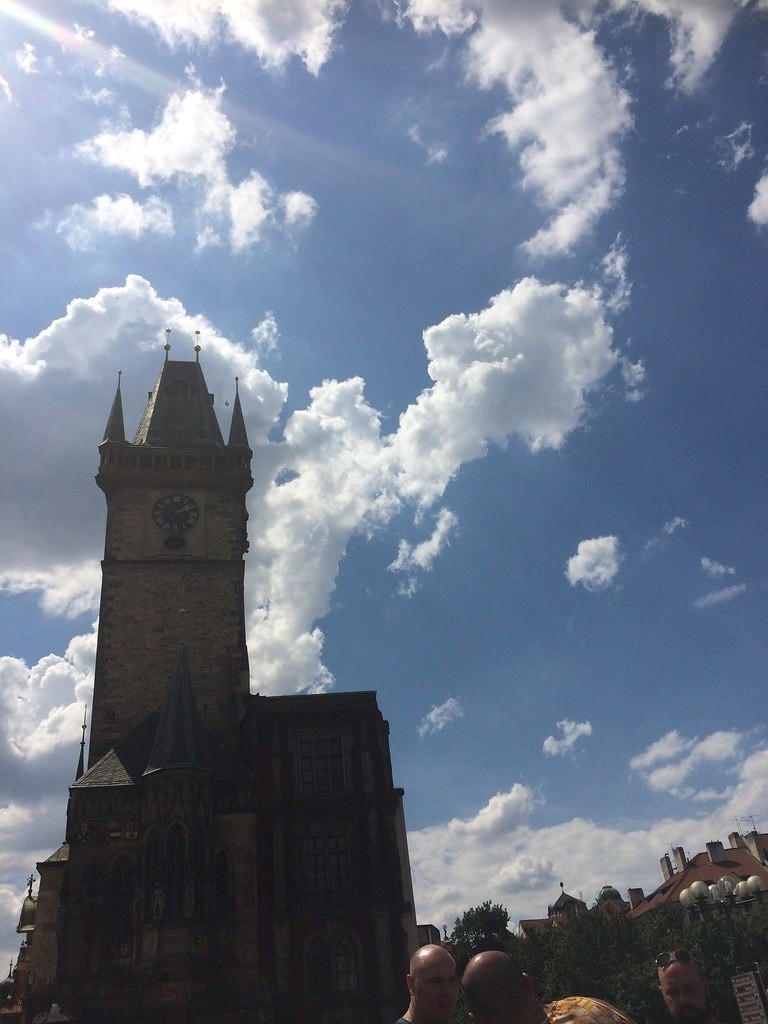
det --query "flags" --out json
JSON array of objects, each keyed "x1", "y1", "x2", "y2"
[{"x1": 225, "y1": 400, "x2": 229, "y2": 407}]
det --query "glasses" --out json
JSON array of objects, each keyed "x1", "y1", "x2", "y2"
[{"x1": 654, "y1": 950, "x2": 701, "y2": 970}]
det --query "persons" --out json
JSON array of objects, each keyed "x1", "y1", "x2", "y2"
[
  {"x1": 453, "y1": 945, "x2": 637, "y2": 1024},
  {"x1": 394, "y1": 944, "x2": 458, "y2": 1024},
  {"x1": 654, "y1": 950, "x2": 716, "y2": 1024}
]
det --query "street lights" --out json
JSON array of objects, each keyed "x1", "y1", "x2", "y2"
[
  {"x1": 443, "y1": 925, "x2": 448, "y2": 941},
  {"x1": 20, "y1": 971, "x2": 36, "y2": 1023},
  {"x1": 679, "y1": 875, "x2": 765, "y2": 972},
  {"x1": 28, "y1": 876, "x2": 36, "y2": 896}
]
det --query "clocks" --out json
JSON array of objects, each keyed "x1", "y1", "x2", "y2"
[{"x1": 153, "y1": 494, "x2": 200, "y2": 532}]
